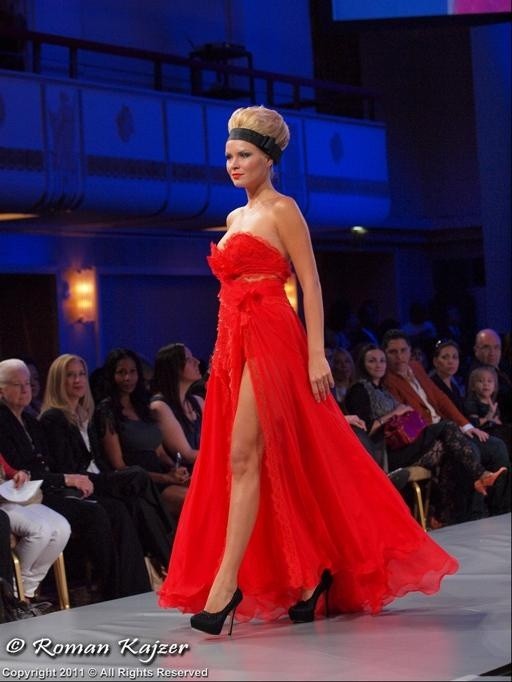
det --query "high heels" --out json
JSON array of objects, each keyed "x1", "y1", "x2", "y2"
[
  {"x1": 288, "y1": 569, "x2": 333, "y2": 623},
  {"x1": 190, "y1": 586, "x2": 243, "y2": 635},
  {"x1": 475, "y1": 467, "x2": 507, "y2": 495}
]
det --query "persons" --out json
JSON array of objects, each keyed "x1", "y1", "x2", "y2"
[
  {"x1": 153, "y1": 101, "x2": 464, "y2": 639},
  {"x1": 0, "y1": 327, "x2": 512, "y2": 626}
]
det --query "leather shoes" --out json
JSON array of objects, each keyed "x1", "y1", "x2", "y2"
[{"x1": 387, "y1": 468, "x2": 410, "y2": 485}]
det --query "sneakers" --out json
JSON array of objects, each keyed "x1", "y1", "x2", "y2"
[
  {"x1": 11, "y1": 598, "x2": 34, "y2": 622},
  {"x1": 25, "y1": 595, "x2": 52, "y2": 617}
]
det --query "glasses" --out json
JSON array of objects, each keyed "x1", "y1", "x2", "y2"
[{"x1": 1, "y1": 381, "x2": 31, "y2": 387}]
